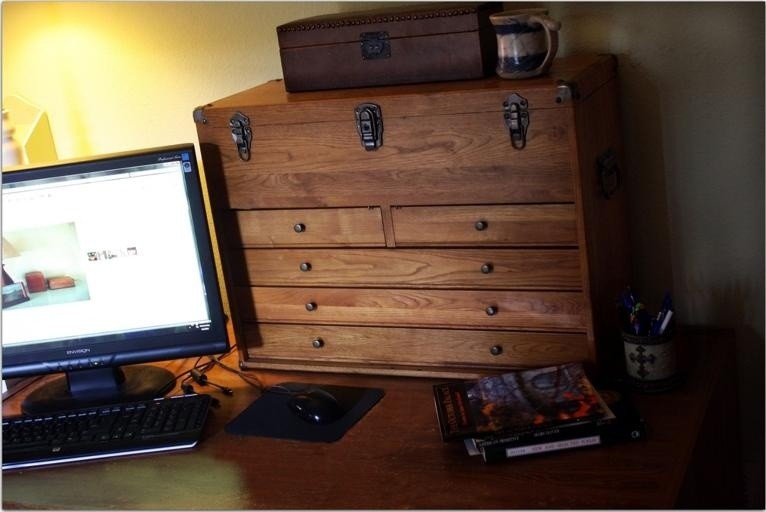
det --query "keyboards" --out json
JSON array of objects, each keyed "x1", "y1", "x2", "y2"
[{"x1": 2, "y1": 393, "x2": 212, "y2": 470}]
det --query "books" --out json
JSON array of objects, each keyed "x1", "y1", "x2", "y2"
[{"x1": 431, "y1": 361, "x2": 618, "y2": 462}]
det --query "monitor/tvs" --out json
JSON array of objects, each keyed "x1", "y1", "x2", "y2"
[{"x1": 1, "y1": 141, "x2": 232, "y2": 416}]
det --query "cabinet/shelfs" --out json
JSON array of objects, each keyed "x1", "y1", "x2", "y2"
[{"x1": 192, "y1": 50, "x2": 637, "y2": 388}]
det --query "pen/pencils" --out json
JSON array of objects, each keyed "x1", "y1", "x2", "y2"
[{"x1": 616, "y1": 285, "x2": 675, "y2": 337}]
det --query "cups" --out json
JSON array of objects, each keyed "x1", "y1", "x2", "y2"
[{"x1": 489, "y1": 8, "x2": 561, "y2": 79}]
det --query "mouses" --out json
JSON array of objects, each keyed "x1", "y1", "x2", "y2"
[{"x1": 285, "y1": 386, "x2": 343, "y2": 425}]
